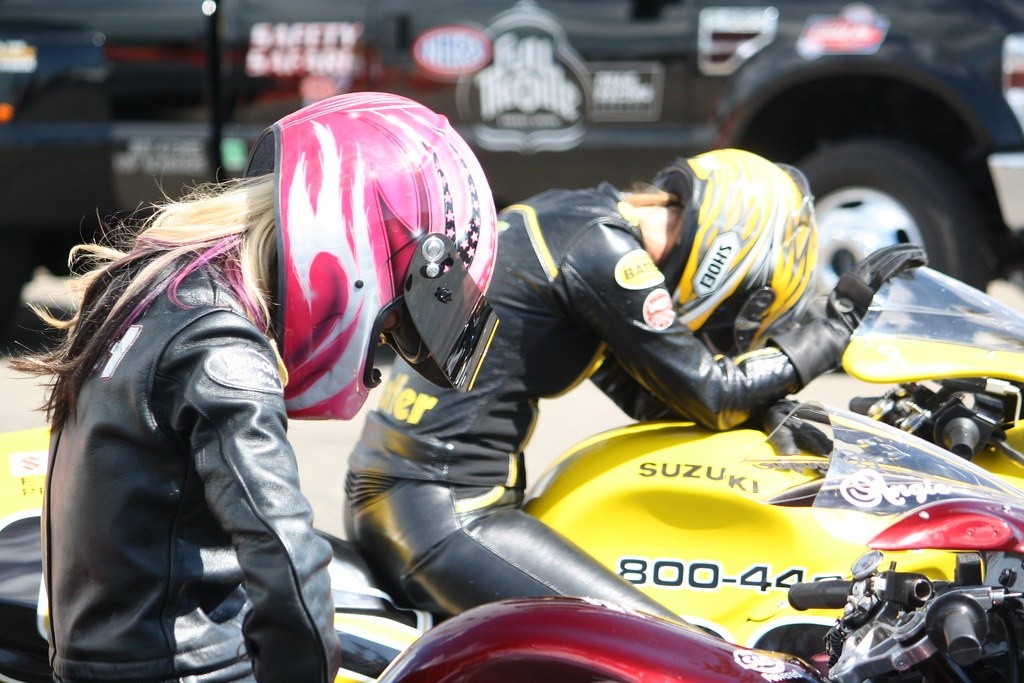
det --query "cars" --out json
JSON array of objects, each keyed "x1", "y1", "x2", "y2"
[{"x1": 1, "y1": 0, "x2": 1021, "y2": 352}]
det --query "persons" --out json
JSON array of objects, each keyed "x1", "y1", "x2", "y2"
[
  {"x1": 344, "y1": 147, "x2": 928, "y2": 646},
  {"x1": 7, "y1": 90, "x2": 500, "y2": 682}
]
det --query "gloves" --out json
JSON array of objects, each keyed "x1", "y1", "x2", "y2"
[{"x1": 767, "y1": 242, "x2": 927, "y2": 382}]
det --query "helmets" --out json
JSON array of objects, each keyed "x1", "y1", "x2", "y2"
[
  {"x1": 652, "y1": 149, "x2": 820, "y2": 359},
  {"x1": 241, "y1": 89, "x2": 499, "y2": 422}
]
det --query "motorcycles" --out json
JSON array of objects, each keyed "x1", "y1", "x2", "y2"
[{"x1": 0, "y1": 242, "x2": 1024, "y2": 681}]
